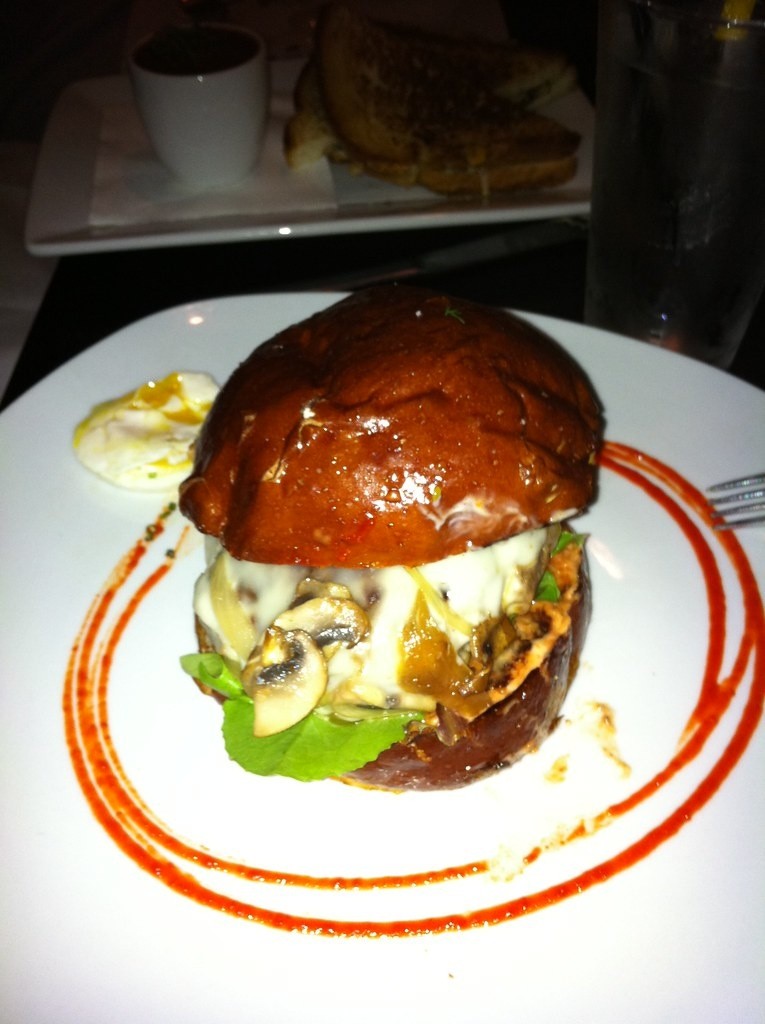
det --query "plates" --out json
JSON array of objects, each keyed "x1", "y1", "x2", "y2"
[
  {"x1": 22, "y1": 56, "x2": 598, "y2": 257},
  {"x1": 1, "y1": 290, "x2": 765, "y2": 1023}
]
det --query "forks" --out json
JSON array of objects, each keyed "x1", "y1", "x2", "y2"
[{"x1": 704, "y1": 471, "x2": 765, "y2": 535}]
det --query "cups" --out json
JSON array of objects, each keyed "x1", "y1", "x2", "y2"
[
  {"x1": 583, "y1": 0, "x2": 765, "y2": 372},
  {"x1": 126, "y1": 21, "x2": 274, "y2": 193}
]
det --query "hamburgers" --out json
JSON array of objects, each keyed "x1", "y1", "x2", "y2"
[{"x1": 180, "y1": 289, "x2": 607, "y2": 789}]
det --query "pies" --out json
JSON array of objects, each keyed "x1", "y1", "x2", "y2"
[{"x1": 283, "y1": 12, "x2": 580, "y2": 194}]
